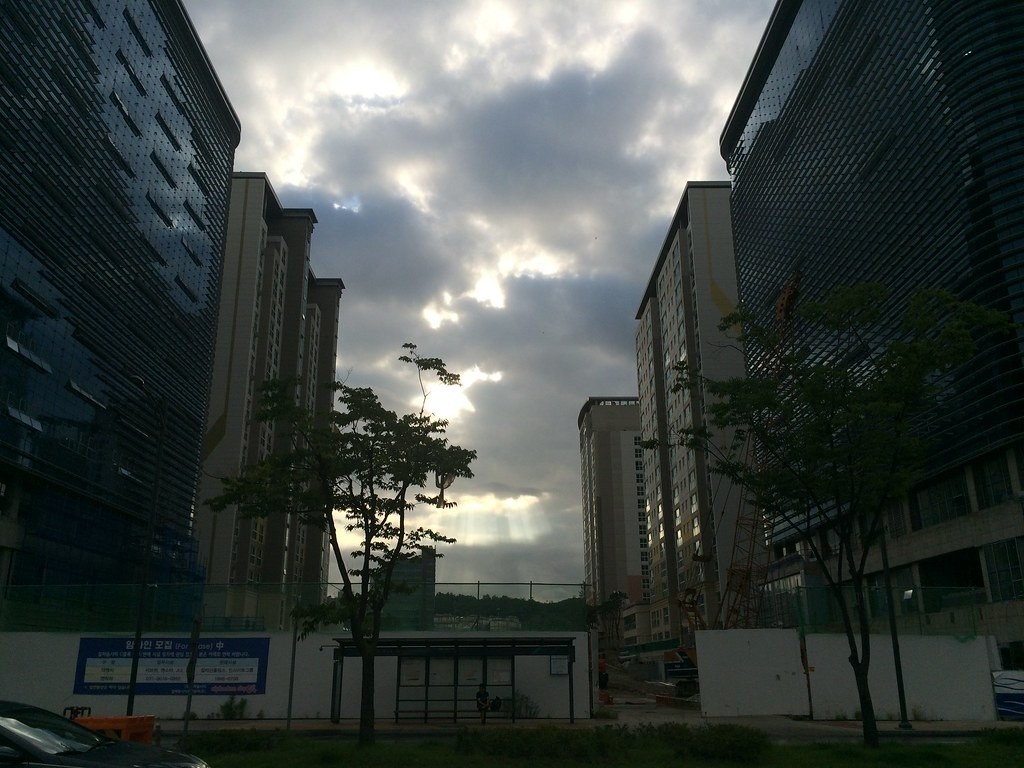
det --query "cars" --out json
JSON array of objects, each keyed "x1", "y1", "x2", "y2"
[{"x1": 0, "y1": 699, "x2": 213, "y2": 768}]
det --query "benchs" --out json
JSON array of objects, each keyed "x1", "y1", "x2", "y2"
[{"x1": 394, "y1": 710, "x2": 515, "y2": 725}]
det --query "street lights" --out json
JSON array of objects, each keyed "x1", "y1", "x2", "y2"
[{"x1": 121, "y1": 371, "x2": 164, "y2": 716}]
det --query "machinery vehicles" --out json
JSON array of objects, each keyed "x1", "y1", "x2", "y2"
[{"x1": 663, "y1": 281, "x2": 805, "y2": 699}]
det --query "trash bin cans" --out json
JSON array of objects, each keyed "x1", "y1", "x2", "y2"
[{"x1": 75, "y1": 715, "x2": 155, "y2": 745}]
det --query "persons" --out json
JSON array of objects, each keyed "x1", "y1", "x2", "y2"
[{"x1": 477, "y1": 684, "x2": 490, "y2": 724}]
